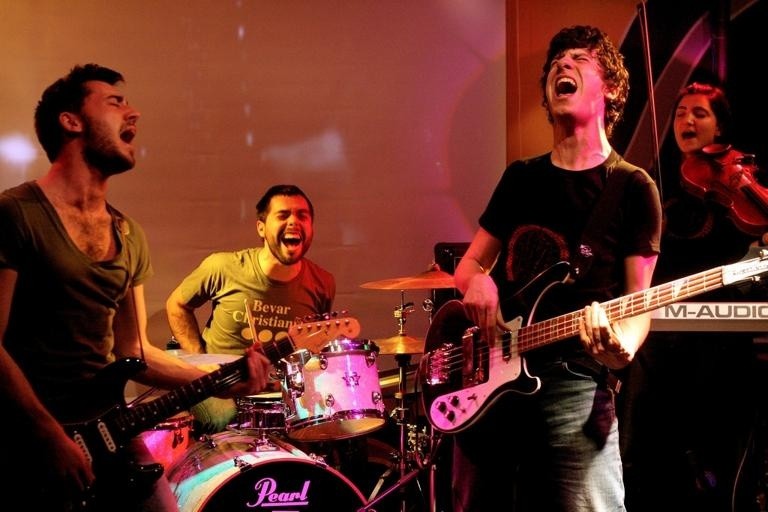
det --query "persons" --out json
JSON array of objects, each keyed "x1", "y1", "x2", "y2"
[
  {"x1": 648, "y1": 83, "x2": 767, "y2": 305},
  {"x1": 453, "y1": 26, "x2": 668, "y2": 512},
  {"x1": 165, "y1": 184, "x2": 338, "y2": 355},
  {"x1": 2, "y1": 63, "x2": 275, "y2": 512}
]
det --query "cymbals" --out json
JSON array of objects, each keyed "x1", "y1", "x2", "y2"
[
  {"x1": 176, "y1": 354, "x2": 244, "y2": 372},
  {"x1": 359, "y1": 270, "x2": 459, "y2": 290},
  {"x1": 374, "y1": 334, "x2": 426, "y2": 354},
  {"x1": 380, "y1": 361, "x2": 423, "y2": 397}
]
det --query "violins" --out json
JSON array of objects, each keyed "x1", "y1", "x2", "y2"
[{"x1": 681, "y1": 143, "x2": 768, "y2": 236}]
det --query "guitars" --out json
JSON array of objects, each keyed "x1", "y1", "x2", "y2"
[
  {"x1": 420, "y1": 248, "x2": 768, "y2": 434},
  {"x1": 0, "y1": 311, "x2": 360, "y2": 512}
]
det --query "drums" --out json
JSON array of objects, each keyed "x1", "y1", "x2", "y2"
[
  {"x1": 165, "y1": 428, "x2": 377, "y2": 512},
  {"x1": 237, "y1": 389, "x2": 287, "y2": 440},
  {"x1": 268, "y1": 338, "x2": 389, "y2": 445},
  {"x1": 131, "y1": 413, "x2": 192, "y2": 479}
]
